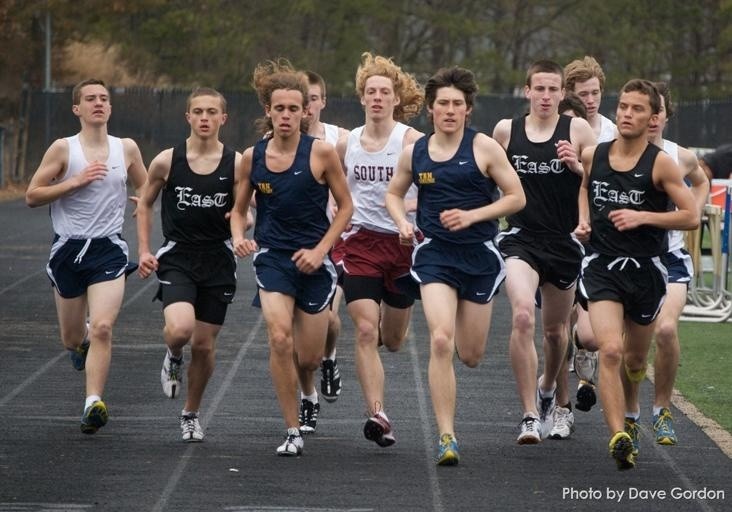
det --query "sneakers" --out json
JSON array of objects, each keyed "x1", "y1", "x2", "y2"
[
  {"x1": 568, "y1": 323, "x2": 600, "y2": 412},
  {"x1": 434, "y1": 431, "x2": 461, "y2": 467},
  {"x1": 66, "y1": 322, "x2": 92, "y2": 371},
  {"x1": 608, "y1": 412, "x2": 643, "y2": 471},
  {"x1": 276, "y1": 427, "x2": 304, "y2": 456},
  {"x1": 80, "y1": 400, "x2": 109, "y2": 436},
  {"x1": 363, "y1": 410, "x2": 397, "y2": 449},
  {"x1": 320, "y1": 358, "x2": 342, "y2": 404},
  {"x1": 160, "y1": 347, "x2": 185, "y2": 400},
  {"x1": 652, "y1": 406, "x2": 679, "y2": 446},
  {"x1": 179, "y1": 409, "x2": 206, "y2": 443},
  {"x1": 516, "y1": 374, "x2": 575, "y2": 446},
  {"x1": 298, "y1": 395, "x2": 321, "y2": 435}
]
